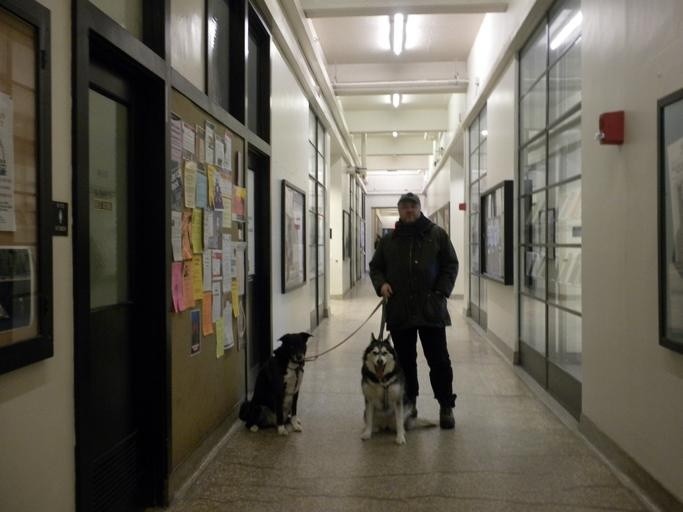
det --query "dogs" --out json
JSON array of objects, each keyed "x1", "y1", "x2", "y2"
[
  {"x1": 238, "y1": 332, "x2": 313, "y2": 436},
  {"x1": 360, "y1": 333, "x2": 414, "y2": 449}
]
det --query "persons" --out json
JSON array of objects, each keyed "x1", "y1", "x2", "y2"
[{"x1": 370, "y1": 193, "x2": 458, "y2": 430}]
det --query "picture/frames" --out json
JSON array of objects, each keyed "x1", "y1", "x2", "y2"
[
  {"x1": 280, "y1": 179, "x2": 309, "y2": 294},
  {"x1": 479, "y1": 177, "x2": 512, "y2": 285},
  {"x1": 0, "y1": 2, "x2": 56, "y2": 374},
  {"x1": 657, "y1": 87, "x2": 683, "y2": 356},
  {"x1": 342, "y1": 209, "x2": 351, "y2": 261}
]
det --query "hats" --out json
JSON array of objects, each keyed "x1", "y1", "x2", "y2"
[{"x1": 397, "y1": 192, "x2": 422, "y2": 205}]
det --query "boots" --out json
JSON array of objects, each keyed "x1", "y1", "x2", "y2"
[
  {"x1": 409, "y1": 401, "x2": 418, "y2": 418},
  {"x1": 439, "y1": 404, "x2": 456, "y2": 429}
]
